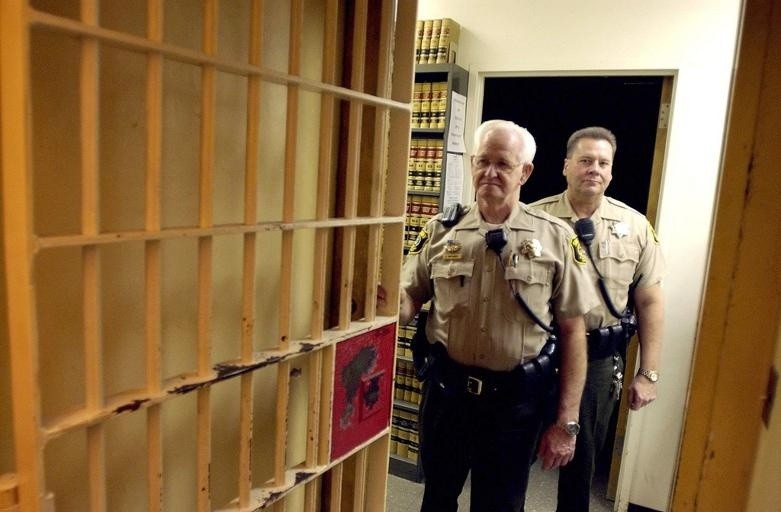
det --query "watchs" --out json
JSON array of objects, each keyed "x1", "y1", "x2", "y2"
[
  {"x1": 556, "y1": 418, "x2": 580, "y2": 436},
  {"x1": 637, "y1": 367, "x2": 660, "y2": 382}
]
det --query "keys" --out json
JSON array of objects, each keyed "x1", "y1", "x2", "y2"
[{"x1": 610, "y1": 371, "x2": 623, "y2": 402}]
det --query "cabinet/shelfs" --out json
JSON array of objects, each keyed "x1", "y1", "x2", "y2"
[{"x1": 387, "y1": 63, "x2": 470, "y2": 484}]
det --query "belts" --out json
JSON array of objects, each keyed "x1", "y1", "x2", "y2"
[{"x1": 434, "y1": 360, "x2": 525, "y2": 398}]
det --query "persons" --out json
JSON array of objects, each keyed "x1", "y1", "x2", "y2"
[
  {"x1": 527, "y1": 127, "x2": 663, "y2": 512},
  {"x1": 399, "y1": 119, "x2": 599, "y2": 512}
]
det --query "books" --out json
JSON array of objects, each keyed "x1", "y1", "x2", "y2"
[{"x1": 389, "y1": 18, "x2": 460, "y2": 462}]
